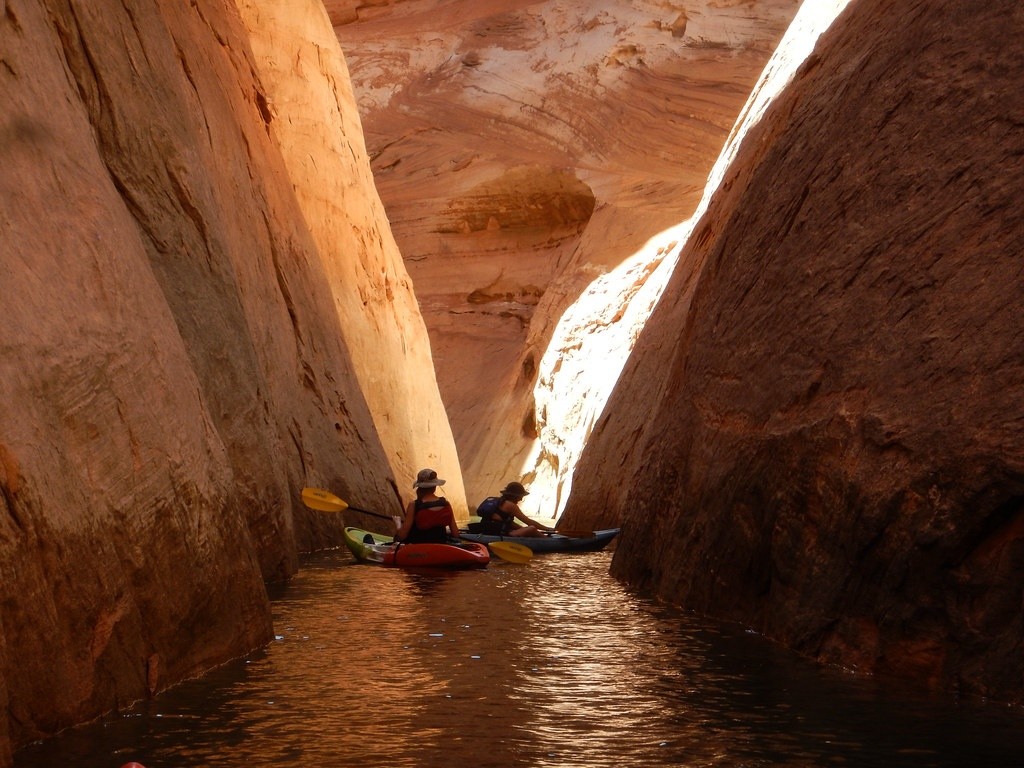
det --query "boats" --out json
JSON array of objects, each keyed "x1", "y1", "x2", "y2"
[
  {"x1": 342, "y1": 524, "x2": 490, "y2": 567},
  {"x1": 456, "y1": 526, "x2": 623, "y2": 553}
]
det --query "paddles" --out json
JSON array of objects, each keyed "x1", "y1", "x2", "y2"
[
  {"x1": 457, "y1": 525, "x2": 598, "y2": 541},
  {"x1": 301, "y1": 486, "x2": 534, "y2": 564}
]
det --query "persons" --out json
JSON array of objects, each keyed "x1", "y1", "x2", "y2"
[
  {"x1": 390, "y1": 469, "x2": 460, "y2": 541},
  {"x1": 480, "y1": 481, "x2": 554, "y2": 537}
]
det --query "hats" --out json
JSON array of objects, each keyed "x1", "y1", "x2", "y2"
[
  {"x1": 413, "y1": 469, "x2": 446, "y2": 487},
  {"x1": 500, "y1": 482, "x2": 530, "y2": 496}
]
